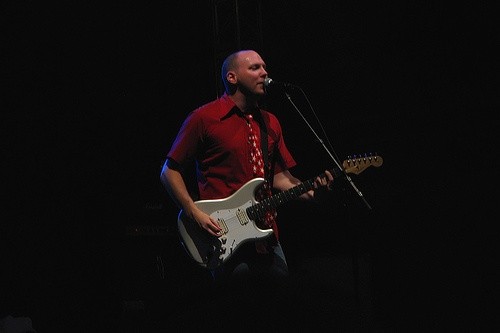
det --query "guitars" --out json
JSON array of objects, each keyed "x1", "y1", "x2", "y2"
[{"x1": 177, "y1": 152, "x2": 384, "y2": 270}]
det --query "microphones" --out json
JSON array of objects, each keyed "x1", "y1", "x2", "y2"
[{"x1": 264, "y1": 78, "x2": 301, "y2": 89}]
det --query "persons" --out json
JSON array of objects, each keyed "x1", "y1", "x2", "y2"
[{"x1": 160, "y1": 50, "x2": 334, "y2": 279}]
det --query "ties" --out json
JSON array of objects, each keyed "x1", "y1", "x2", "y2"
[{"x1": 244, "y1": 113, "x2": 275, "y2": 229}]
